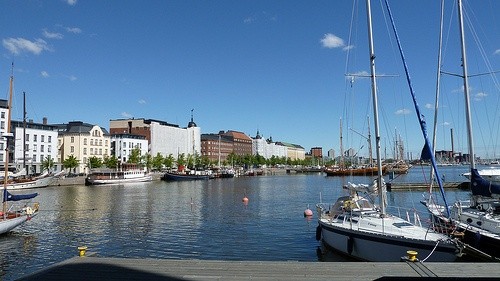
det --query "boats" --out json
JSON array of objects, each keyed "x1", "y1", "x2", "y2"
[
  {"x1": 0, "y1": 167, "x2": 67, "y2": 190},
  {"x1": 86, "y1": 165, "x2": 153, "y2": 185},
  {"x1": 164, "y1": 163, "x2": 236, "y2": 181}
]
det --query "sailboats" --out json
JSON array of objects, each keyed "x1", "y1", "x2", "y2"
[
  {"x1": 314, "y1": 0, "x2": 467, "y2": 262},
  {"x1": 325, "y1": 117, "x2": 408, "y2": 175},
  {"x1": 420, "y1": 0, "x2": 499, "y2": 262},
  {"x1": 0, "y1": 61, "x2": 40, "y2": 236}
]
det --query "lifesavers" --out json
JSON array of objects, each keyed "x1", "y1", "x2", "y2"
[
  {"x1": 316, "y1": 226, "x2": 322, "y2": 241},
  {"x1": 347, "y1": 238, "x2": 354, "y2": 253}
]
